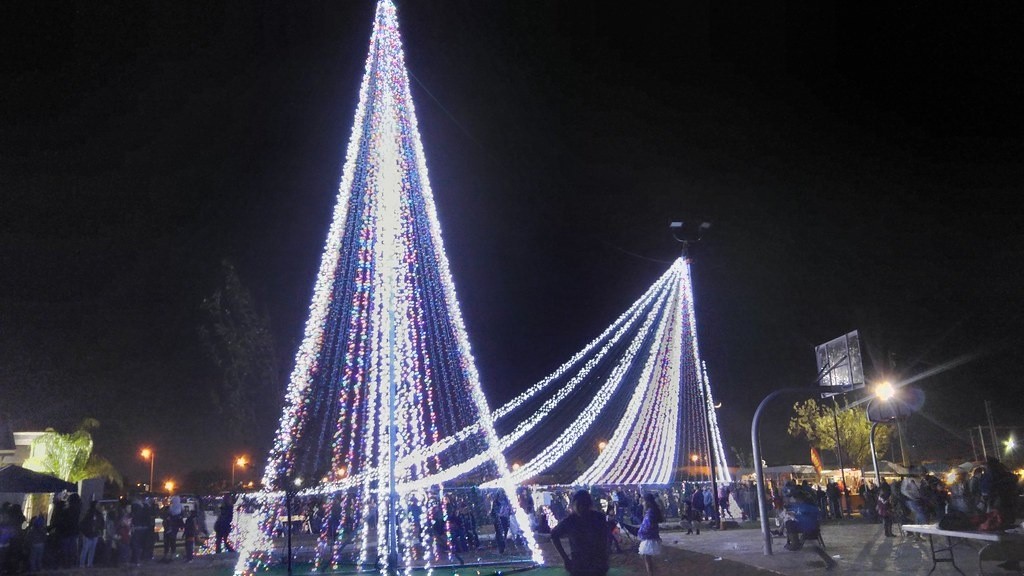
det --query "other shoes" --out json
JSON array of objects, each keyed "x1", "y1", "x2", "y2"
[
  {"x1": 687, "y1": 528, "x2": 693, "y2": 535},
  {"x1": 188, "y1": 559, "x2": 194, "y2": 564},
  {"x1": 915, "y1": 536, "x2": 927, "y2": 543},
  {"x1": 771, "y1": 529, "x2": 783, "y2": 537},
  {"x1": 131, "y1": 563, "x2": 143, "y2": 568},
  {"x1": 615, "y1": 548, "x2": 622, "y2": 554},
  {"x1": 887, "y1": 532, "x2": 897, "y2": 537},
  {"x1": 784, "y1": 543, "x2": 790, "y2": 550}
]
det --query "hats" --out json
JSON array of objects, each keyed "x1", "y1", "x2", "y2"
[{"x1": 34, "y1": 517, "x2": 45, "y2": 527}]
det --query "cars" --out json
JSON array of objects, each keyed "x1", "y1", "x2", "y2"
[
  {"x1": 203, "y1": 497, "x2": 223, "y2": 532},
  {"x1": 947, "y1": 456, "x2": 1018, "y2": 523},
  {"x1": 176, "y1": 493, "x2": 197, "y2": 511}
]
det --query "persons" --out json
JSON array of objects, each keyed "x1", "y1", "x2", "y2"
[
  {"x1": 446, "y1": 486, "x2": 534, "y2": 552},
  {"x1": 0, "y1": 492, "x2": 199, "y2": 572},
  {"x1": 538, "y1": 489, "x2": 677, "y2": 576},
  {"x1": 733, "y1": 477, "x2": 843, "y2": 550},
  {"x1": 717, "y1": 482, "x2": 732, "y2": 517},
  {"x1": 215, "y1": 494, "x2": 234, "y2": 553},
  {"x1": 858, "y1": 457, "x2": 1024, "y2": 568},
  {"x1": 682, "y1": 482, "x2": 711, "y2": 535}
]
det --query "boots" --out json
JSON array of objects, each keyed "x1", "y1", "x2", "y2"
[{"x1": 788, "y1": 532, "x2": 799, "y2": 551}]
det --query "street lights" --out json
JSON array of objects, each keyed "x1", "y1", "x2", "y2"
[
  {"x1": 231, "y1": 455, "x2": 247, "y2": 491},
  {"x1": 165, "y1": 481, "x2": 174, "y2": 496},
  {"x1": 142, "y1": 449, "x2": 153, "y2": 492},
  {"x1": 598, "y1": 441, "x2": 606, "y2": 453},
  {"x1": 1006, "y1": 440, "x2": 1023, "y2": 468},
  {"x1": 692, "y1": 455, "x2": 699, "y2": 482}
]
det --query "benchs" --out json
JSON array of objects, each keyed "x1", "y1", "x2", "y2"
[
  {"x1": 797, "y1": 522, "x2": 824, "y2": 550},
  {"x1": 902, "y1": 524, "x2": 1024, "y2": 576}
]
